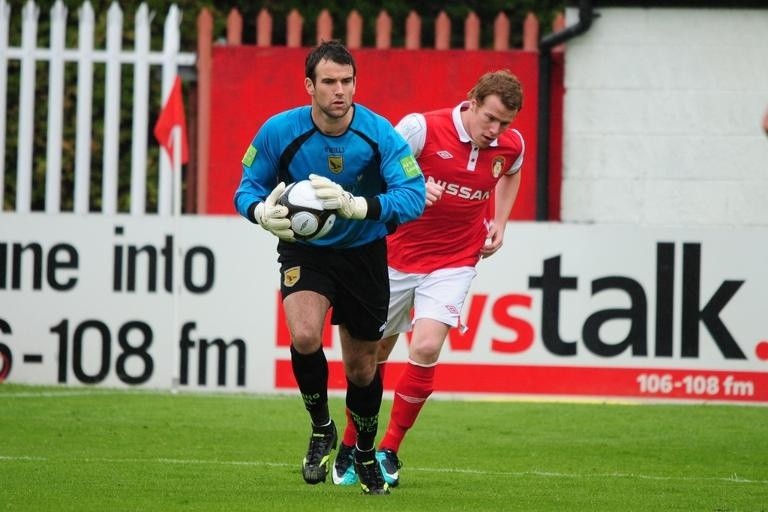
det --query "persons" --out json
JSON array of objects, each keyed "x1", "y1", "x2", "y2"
[
  {"x1": 231, "y1": 38, "x2": 425, "y2": 497},
  {"x1": 332, "y1": 67, "x2": 525, "y2": 486}
]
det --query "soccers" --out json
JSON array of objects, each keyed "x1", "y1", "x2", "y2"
[{"x1": 275, "y1": 179, "x2": 337, "y2": 242}]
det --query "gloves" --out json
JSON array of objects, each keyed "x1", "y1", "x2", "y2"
[
  {"x1": 307, "y1": 173, "x2": 368, "y2": 220},
  {"x1": 253, "y1": 182, "x2": 296, "y2": 244}
]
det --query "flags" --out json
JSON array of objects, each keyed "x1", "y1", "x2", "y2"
[{"x1": 153, "y1": 74, "x2": 189, "y2": 169}]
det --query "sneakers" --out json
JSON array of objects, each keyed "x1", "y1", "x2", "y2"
[
  {"x1": 374, "y1": 448, "x2": 403, "y2": 487},
  {"x1": 331, "y1": 446, "x2": 358, "y2": 486},
  {"x1": 301, "y1": 420, "x2": 337, "y2": 485},
  {"x1": 352, "y1": 448, "x2": 390, "y2": 495}
]
